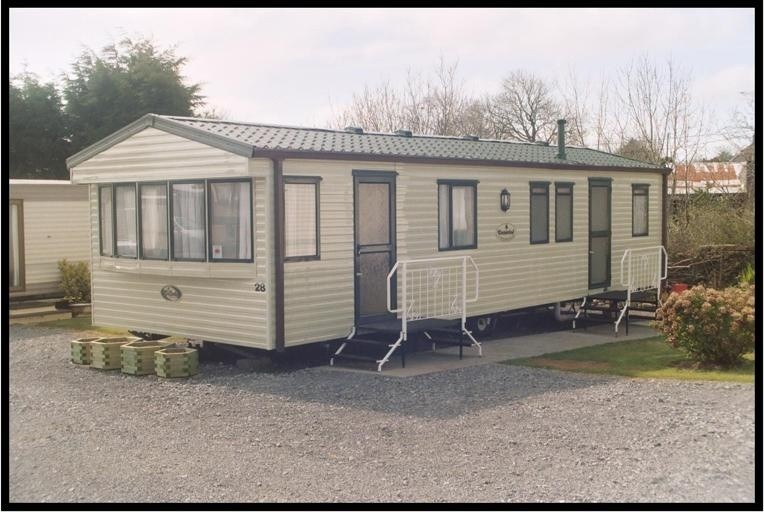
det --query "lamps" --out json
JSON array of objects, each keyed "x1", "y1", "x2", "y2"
[{"x1": 500, "y1": 187, "x2": 511, "y2": 213}]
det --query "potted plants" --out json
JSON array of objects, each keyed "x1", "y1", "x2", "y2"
[{"x1": 58, "y1": 255, "x2": 91, "y2": 319}]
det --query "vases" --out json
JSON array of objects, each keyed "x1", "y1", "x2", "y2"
[{"x1": 70, "y1": 336, "x2": 198, "y2": 378}]
